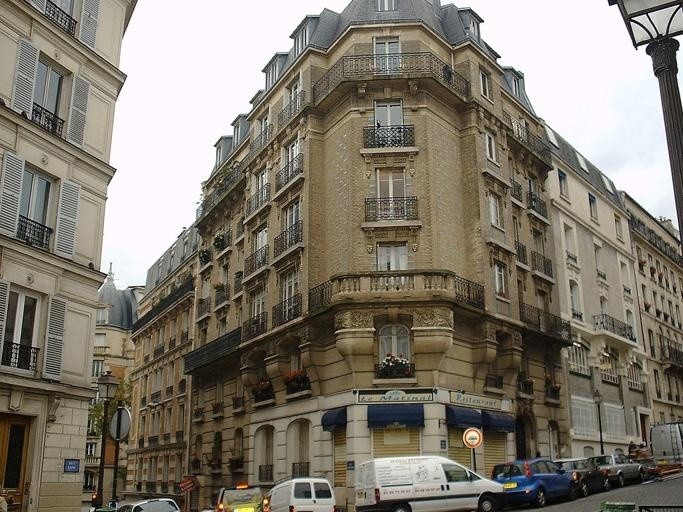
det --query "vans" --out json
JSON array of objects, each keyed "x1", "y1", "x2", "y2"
[
  {"x1": 197, "y1": 477, "x2": 337, "y2": 512},
  {"x1": 354, "y1": 455, "x2": 504, "y2": 512},
  {"x1": 116, "y1": 498, "x2": 182, "y2": 512}
]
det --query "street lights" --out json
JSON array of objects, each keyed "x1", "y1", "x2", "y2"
[
  {"x1": 592, "y1": 389, "x2": 605, "y2": 455},
  {"x1": 97, "y1": 370, "x2": 120, "y2": 508},
  {"x1": 606, "y1": 1, "x2": 683, "y2": 260}
]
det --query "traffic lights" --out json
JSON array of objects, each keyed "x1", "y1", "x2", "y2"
[{"x1": 92, "y1": 492, "x2": 97, "y2": 508}]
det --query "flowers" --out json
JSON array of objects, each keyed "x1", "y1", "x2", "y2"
[
  {"x1": 198, "y1": 232, "x2": 224, "y2": 262},
  {"x1": 252, "y1": 375, "x2": 273, "y2": 394},
  {"x1": 379, "y1": 352, "x2": 413, "y2": 377},
  {"x1": 519, "y1": 370, "x2": 536, "y2": 386},
  {"x1": 544, "y1": 372, "x2": 561, "y2": 393},
  {"x1": 284, "y1": 369, "x2": 308, "y2": 385}
]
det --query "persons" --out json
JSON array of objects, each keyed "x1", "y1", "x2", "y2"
[{"x1": 629, "y1": 441, "x2": 637, "y2": 463}]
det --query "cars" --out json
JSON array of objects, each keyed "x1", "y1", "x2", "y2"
[{"x1": 491, "y1": 454, "x2": 645, "y2": 507}]
